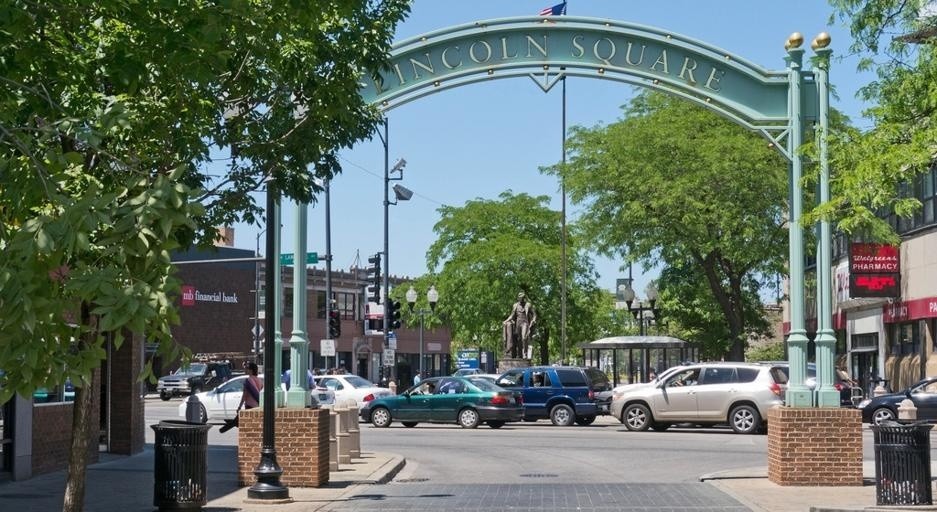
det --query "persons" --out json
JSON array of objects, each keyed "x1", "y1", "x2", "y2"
[
  {"x1": 280, "y1": 368, "x2": 316, "y2": 391},
  {"x1": 236, "y1": 361, "x2": 261, "y2": 414},
  {"x1": 533, "y1": 374, "x2": 542, "y2": 386},
  {"x1": 501, "y1": 376, "x2": 523, "y2": 386},
  {"x1": 503, "y1": 292, "x2": 537, "y2": 359},
  {"x1": 416, "y1": 382, "x2": 434, "y2": 395},
  {"x1": 325, "y1": 366, "x2": 345, "y2": 377},
  {"x1": 413, "y1": 369, "x2": 421, "y2": 386},
  {"x1": 841, "y1": 367, "x2": 858, "y2": 387},
  {"x1": 675, "y1": 373, "x2": 698, "y2": 387}
]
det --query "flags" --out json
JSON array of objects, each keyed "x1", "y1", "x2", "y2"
[{"x1": 537, "y1": 1, "x2": 566, "y2": 18}]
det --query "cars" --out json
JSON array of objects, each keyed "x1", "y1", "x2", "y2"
[
  {"x1": 178, "y1": 374, "x2": 336, "y2": 425},
  {"x1": 857, "y1": 376, "x2": 937, "y2": 426},
  {"x1": 494, "y1": 366, "x2": 614, "y2": 426},
  {"x1": 368, "y1": 368, "x2": 527, "y2": 428},
  {"x1": 313, "y1": 375, "x2": 397, "y2": 422}
]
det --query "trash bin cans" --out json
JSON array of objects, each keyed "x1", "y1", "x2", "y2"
[
  {"x1": 869, "y1": 425, "x2": 935, "y2": 505},
  {"x1": 150, "y1": 424, "x2": 213, "y2": 512}
]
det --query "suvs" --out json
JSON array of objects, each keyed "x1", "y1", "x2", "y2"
[
  {"x1": 155, "y1": 361, "x2": 233, "y2": 401},
  {"x1": 611, "y1": 361, "x2": 852, "y2": 434}
]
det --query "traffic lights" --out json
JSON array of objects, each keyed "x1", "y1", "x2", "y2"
[
  {"x1": 368, "y1": 253, "x2": 381, "y2": 305},
  {"x1": 329, "y1": 309, "x2": 341, "y2": 338},
  {"x1": 387, "y1": 297, "x2": 401, "y2": 331}
]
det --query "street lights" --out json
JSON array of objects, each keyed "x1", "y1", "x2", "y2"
[
  {"x1": 622, "y1": 280, "x2": 669, "y2": 384},
  {"x1": 406, "y1": 284, "x2": 439, "y2": 380}
]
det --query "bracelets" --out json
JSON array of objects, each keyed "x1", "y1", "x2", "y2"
[{"x1": 237, "y1": 407, "x2": 241, "y2": 410}]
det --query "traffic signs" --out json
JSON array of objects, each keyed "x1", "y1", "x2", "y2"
[{"x1": 280, "y1": 253, "x2": 318, "y2": 266}]
